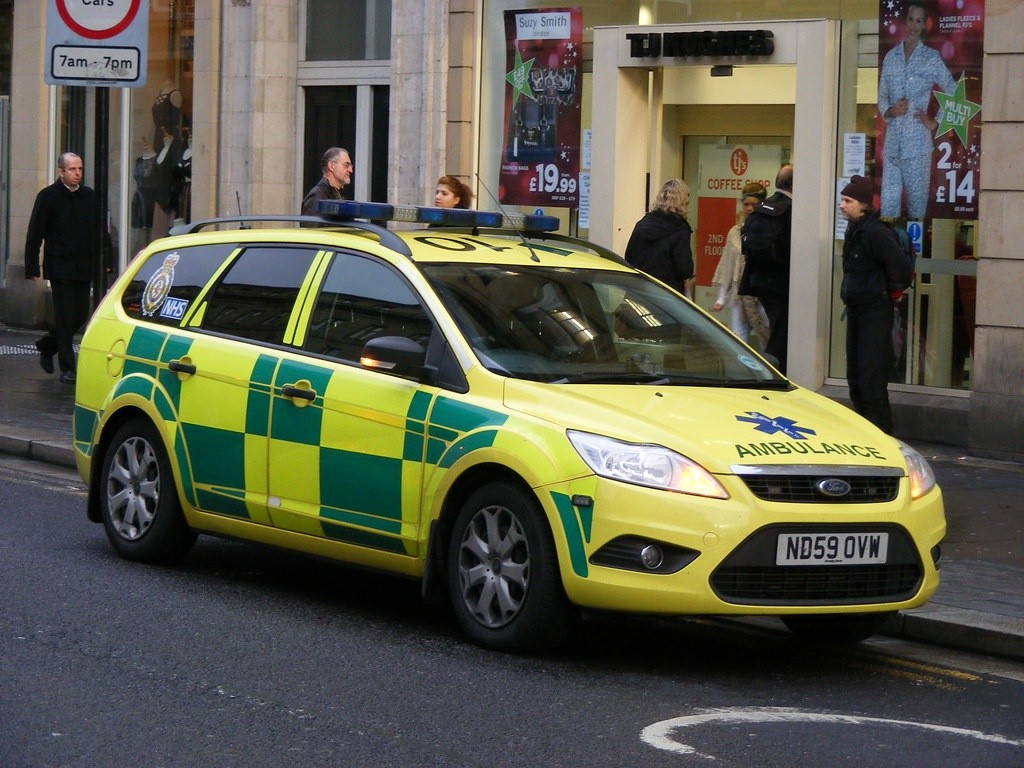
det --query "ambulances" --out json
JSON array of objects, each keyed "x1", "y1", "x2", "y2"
[{"x1": 70, "y1": 201, "x2": 946, "y2": 659}]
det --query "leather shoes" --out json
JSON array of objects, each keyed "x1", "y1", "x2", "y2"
[
  {"x1": 59, "y1": 370, "x2": 76, "y2": 384},
  {"x1": 36, "y1": 335, "x2": 55, "y2": 374}
]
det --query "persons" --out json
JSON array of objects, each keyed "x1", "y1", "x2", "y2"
[
  {"x1": 839, "y1": 182, "x2": 912, "y2": 437},
  {"x1": 876, "y1": 0, "x2": 959, "y2": 222},
  {"x1": 745, "y1": 163, "x2": 794, "y2": 378},
  {"x1": 132, "y1": 144, "x2": 156, "y2": 228},
  {"x1": 178, "y1": 135, "x2": 192, "y2": 224},
  {"x1": 24, "y1": 150, "x2": 113, "y2": 384},
  {"x1": 426, "y1": 174, "x2": 473, "y2": 227},
  {"x1": 624, "y1": 178, "x2": 694, "y2": 299},
  {"x1": 299, "y1": 148, "x2": 352, "y2": 228},
  {"x1": 151, "y1": 79, "x2": 182, "y2": 156},
  {"x1": 151, "y1": 135, "x2": 180, "y2": 242},
  {"x1": 713, "y1": 182, "x2": 771, "y2": 346}
]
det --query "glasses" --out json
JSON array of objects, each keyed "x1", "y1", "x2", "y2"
[{"x1": 328, "y1": 160, "x2": 353, "y2": 169}]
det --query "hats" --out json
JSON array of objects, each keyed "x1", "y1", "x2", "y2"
[{"x1": 841, "y1": 175, "x2": 873, "y2": 205}]
